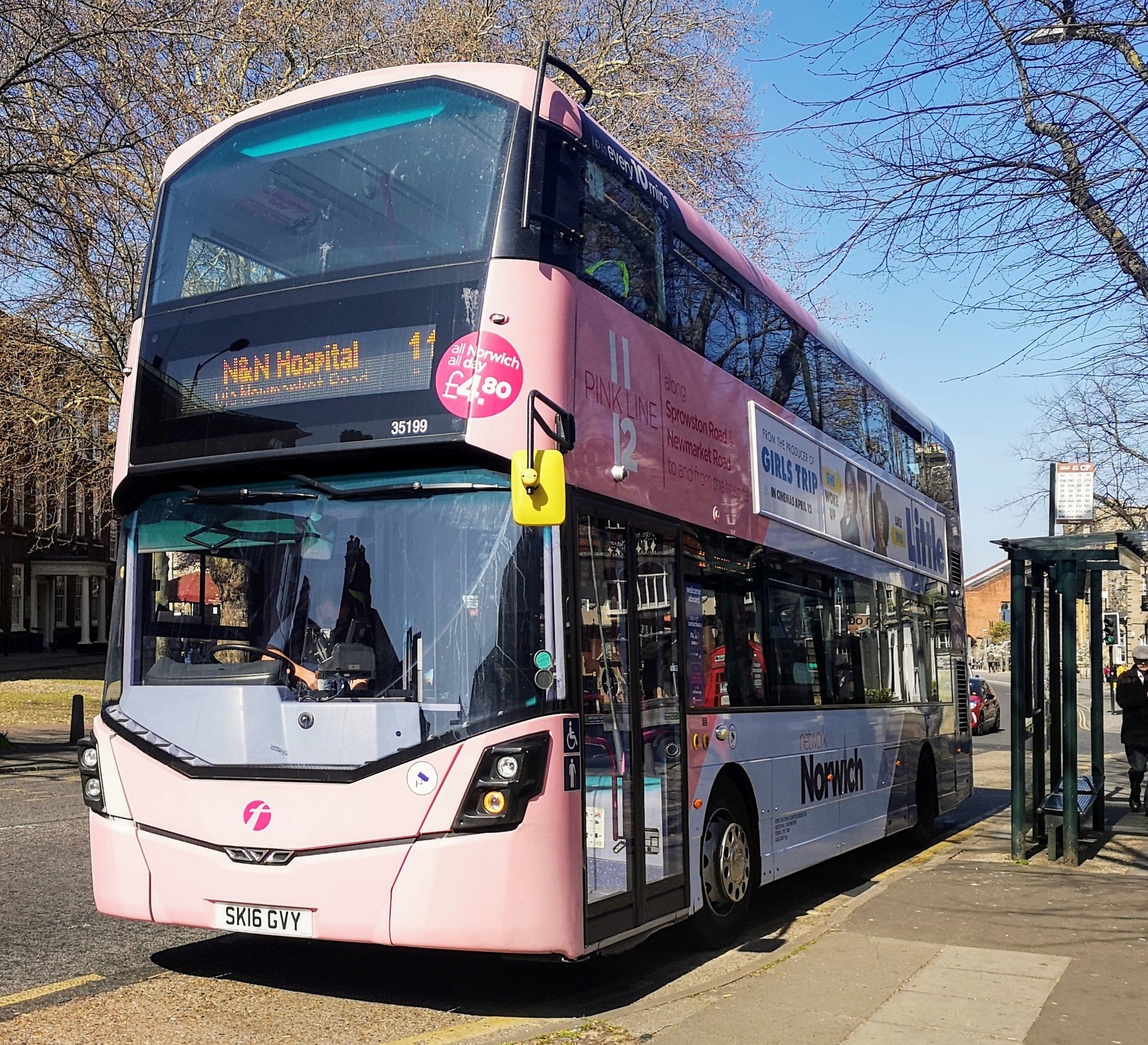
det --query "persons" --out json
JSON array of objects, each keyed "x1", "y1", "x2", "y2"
[
  {"x1": 856, "y1": 468, "x2": 874, "y2": 551},
  {"x1": 1104, "y1": 662, "x2": 1129, "y2": 690},
  {"x1": 262, "y1": 586, "x2": 375, "y2": 692},
  {"x1": 1115, "y1": 646, "x2": 1148, "y2": 816},
  {"x1": 840, "y1": 462, "x2": 861, "y2": 546},
  {"x1": 987, "y1": 651, "x2": 995, "y2": 673},
  {"x1": 868, "y1": 482, "x2": 889, "y2": 557}
]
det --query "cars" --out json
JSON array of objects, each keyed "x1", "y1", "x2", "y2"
[{"x1": 968, "y1": 675, "x2": 1001, "y2": 736}]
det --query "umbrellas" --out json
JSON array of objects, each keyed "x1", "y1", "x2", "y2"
[{"x1": 168, "y1": 571, "x2": 222, "y2": 605}]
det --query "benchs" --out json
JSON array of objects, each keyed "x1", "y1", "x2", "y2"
[{"x1": 1036, "y1": 773, "x2": 1106, "y2": 862}]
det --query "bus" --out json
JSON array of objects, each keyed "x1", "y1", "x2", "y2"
[{"x1": 71, "y1": 39, "x2": 976, "y2": 962}]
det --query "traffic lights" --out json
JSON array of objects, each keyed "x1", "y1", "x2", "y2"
[
  {"x1": 1102, "y1": 612, "x2": 1121, "y2": 646},
  {"x1": 804, "y1": 638, "x2": 818, "y2": 673}
]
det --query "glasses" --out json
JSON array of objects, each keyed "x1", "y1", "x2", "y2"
[{"x1": 319, "y1": 607, "x2": 350, "y2": 626}]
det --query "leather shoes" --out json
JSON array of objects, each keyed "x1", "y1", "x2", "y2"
[{"x1": 1129, "y1": 787, "x2": 1141, "y2": 810}]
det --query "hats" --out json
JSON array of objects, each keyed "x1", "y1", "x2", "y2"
[{"x1": 1132, "y1": 646, "x2": 1148, "y2": 659}]
